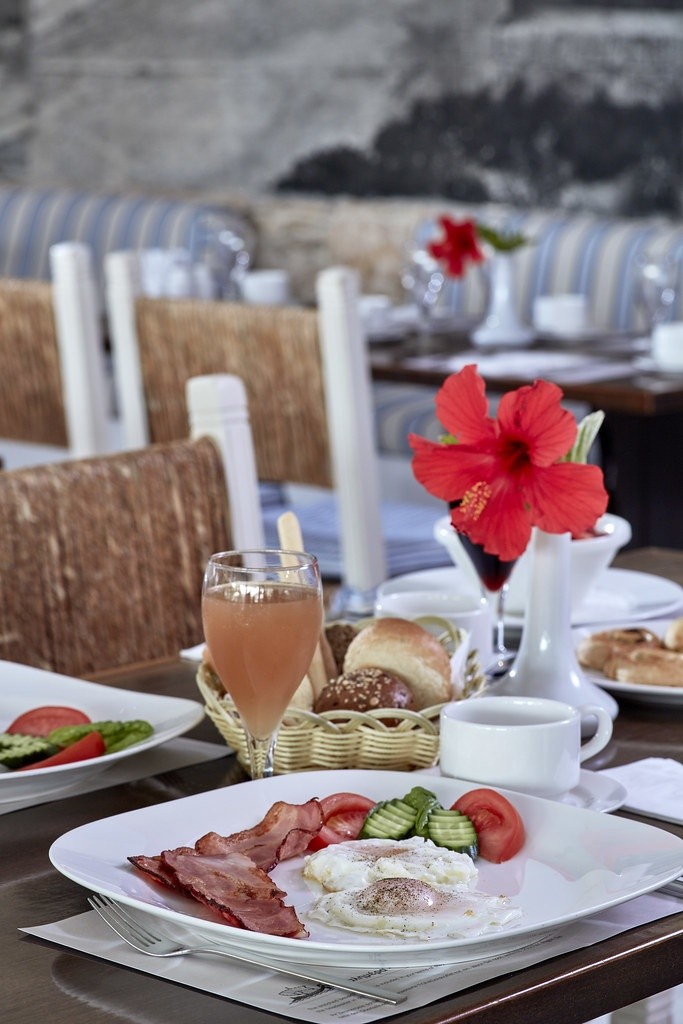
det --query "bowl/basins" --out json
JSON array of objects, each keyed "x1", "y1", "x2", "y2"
[{"x1": 429, "y1": 508, "x2": 632, "y2": 612}]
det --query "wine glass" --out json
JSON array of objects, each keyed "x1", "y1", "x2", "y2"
[
  {"x1": 207, "y1": 227, "x2": 683, "y2": 369},
  {"x1": 445, "y1": 499, "x2": 526, "y2": 678},
  {"x1": 201, "y1": 548, "x2": 325, "y2": 783}
]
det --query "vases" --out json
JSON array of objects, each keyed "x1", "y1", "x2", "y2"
[
  {"x1": 466, "y1": 521, "x2": 619, "y2": 734},
  {"x1": 469, "y1": 252, "x2": 540, "y2": 348}
]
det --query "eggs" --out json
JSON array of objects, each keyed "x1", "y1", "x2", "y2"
[{"x1": 301, "y1": 834, "x2": 521, "y2": 941}]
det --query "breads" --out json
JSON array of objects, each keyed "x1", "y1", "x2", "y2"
[
  {"x1": 575, "y1": 615, "x2": 683, "y2": 690},
  {"x1": 225, "y1": 616, "x2": 453, "y2": 731}
]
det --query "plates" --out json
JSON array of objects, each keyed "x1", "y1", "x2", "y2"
[
  {"x1": 0, "y1": 659, "x2": 205, "y2": 806},
  {"x1": 411, "y1": 766, "x2": 628, "y2": 815},
  {"x1": 567, "y1": 619, "x2": 683, "y2": 706},
  {"x1": 376, "y1": 558, "x2": 683, "y2": 639},
  {"x1": 447, "y1": 351, "x2": 584, "y2": 376},
  {"x1": 48, "y1": 769, "x2": 683, "y2": 971}
]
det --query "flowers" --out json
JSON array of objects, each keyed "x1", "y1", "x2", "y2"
[
  {"x1": 407, "y1": 362, "x2": 608, "y2": 560},
  {"x1": 430, "y1": 212, "x2": 536, "y2": 276}
]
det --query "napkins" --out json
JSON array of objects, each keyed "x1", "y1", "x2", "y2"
[{"x1": 593, "y1": 752, "x2": 683, "y2": 826}]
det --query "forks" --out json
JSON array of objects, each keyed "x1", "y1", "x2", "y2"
[{"x1": 85, "y1": 893, "x2": 409, "y2": 1005}]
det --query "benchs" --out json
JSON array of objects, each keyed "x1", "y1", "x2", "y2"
[{"x1": 0, "y1": 178, "x2": 683, "y2": 674}]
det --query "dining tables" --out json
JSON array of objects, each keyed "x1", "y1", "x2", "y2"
[
  {"x1": 354, "y1": 328, "x2": 683, "y2": 417},
  {"x1": 0, "y1": 541, "x2": 682, "y2": 1024}
]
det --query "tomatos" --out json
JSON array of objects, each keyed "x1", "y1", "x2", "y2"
[
  {"x1": 305, "y1": 793, "x2": 375, "y2": 850},
  {"x1": 5, "y1": 707, "x2": 90, "y2": 737},
  {"x1": 451, "y1": 788, "x2": 524, "y2": 864},
  {"x1": 14, "y1": 729, "x2": 105, "y2": 770}
]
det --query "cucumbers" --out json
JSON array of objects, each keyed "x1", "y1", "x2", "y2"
[
  {"x1": 354, "y1": 799, "x2": 479, "y2": 863},
  {"x1": 0, "y1": 733, "x2": 63, "y2": 768}
]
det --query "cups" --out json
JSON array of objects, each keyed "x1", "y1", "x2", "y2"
[
  {"x1": 536, "y1": 292, "x2": 585, "y2": 331},
  {"x1": 242, "y1": 268, "x2": 290, "y2": 305},
  {"x1": 364, "y1": 294, "x2": 393, "y2": 327},
  {"x1": 652, "y1": 321, "x2": 682, "y2": 373},
  {"x1": 375, "y1": 590, "x2": 491, "y2": 677},
  {"x1": 440, "y1": 693, "x2": 612, "y2": 804},
  {"x1": 143, "y1": 251, "x2": 190, "y2": 298}
]
